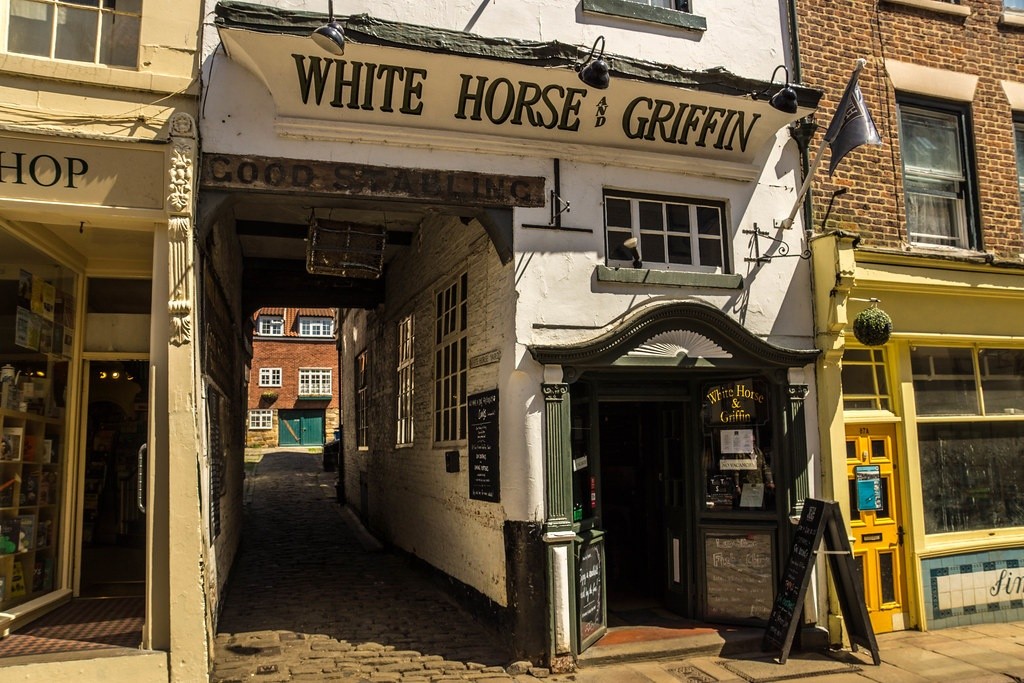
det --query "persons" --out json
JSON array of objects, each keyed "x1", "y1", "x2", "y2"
[{"x1": 732, "y1": 434, "x2": 775, "y2": 493}]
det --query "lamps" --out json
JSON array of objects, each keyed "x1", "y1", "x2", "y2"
[
  {"x1": 751, "y1": 66, "x2": 797, "y2": 114},
  {"x1": 573, "y1": 36, "x2": 610, "y2": 89},
  {"x1": 312, "y1": 16, "x2": 346, "y2": 56}
]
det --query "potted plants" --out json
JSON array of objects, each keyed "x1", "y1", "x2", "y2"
[{"x1": 261, "y1": 392, "x2": 278, "y2": 404}]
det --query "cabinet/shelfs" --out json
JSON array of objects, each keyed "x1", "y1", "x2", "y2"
[{"x1": 0, "y1": 381, "x2": 66, "y2": 613}]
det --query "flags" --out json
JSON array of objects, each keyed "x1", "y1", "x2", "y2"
[{"x1": 822, "y1": 78, "x2": 880, "y2": 179}]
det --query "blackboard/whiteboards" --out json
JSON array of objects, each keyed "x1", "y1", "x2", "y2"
[
  {"x1": 700, "y1": 528, "x2": 777, "y2": 623},
  {"x1": 764, "y1": 496, "x2": 823, "y2": 651},
  {"x1": 577, "y1": 536, "x2": 608, "y2": 655}
]
